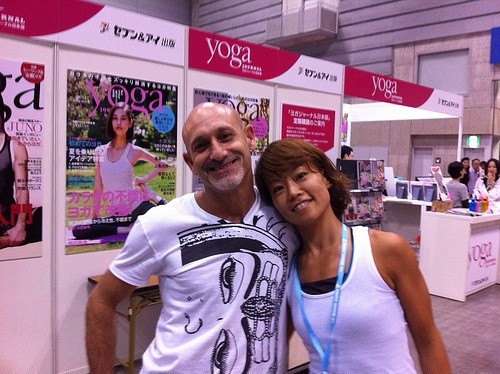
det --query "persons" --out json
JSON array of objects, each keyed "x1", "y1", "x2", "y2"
[
  {"x1": 341, "y1": 146, "x2": 355, "y2": 161},
  {"x1": 256, "y1": 139, "x2": 452, "y2": 374},
  {"x1": 447, "y1": 156, "x2": 500, "y2": 215},
  {"x1": 85, "y1": 102, "x2": 302, "y2": 374},
  {"x1": 0, "y1": 95, "x2": 28, "y2": 251},
  {"x1": 73, "y1": 102, "x2": 169, "y2": 240}
]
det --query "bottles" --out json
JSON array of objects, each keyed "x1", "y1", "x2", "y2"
[{"x1": 470, "y1": 196, "x2": 489, "y2": 213}]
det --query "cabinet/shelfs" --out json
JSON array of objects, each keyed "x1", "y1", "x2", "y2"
[{"x1": 337, "y1": 159, "x2": 384, "y2": 226}]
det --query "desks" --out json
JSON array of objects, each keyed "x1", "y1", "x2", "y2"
[
  {"x1": 86, "y1": 272, "x2": 159, "y2": 374},
  {"x1": 418, "y1": 211, "x2": 500, "y2": 302}
]
